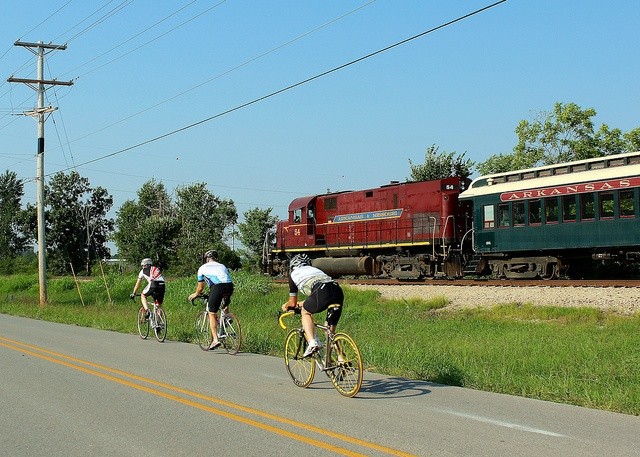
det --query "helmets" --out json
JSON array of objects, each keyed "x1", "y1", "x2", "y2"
[
  {"x1": 140, "y1": 258, "x2": 153, "y2": 266},
  {"x1": 204, "y1": 250, "x2": 217, "y2": 259},
  {"x1": 290, "y1": 253, "x2": 310, "y2": 270}
]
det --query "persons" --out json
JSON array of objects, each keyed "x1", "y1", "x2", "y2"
[
  {"x1": 282, "y1": 253, "x2": 352, "y2": 369},
  {"x1": 131, "y1": 257, "x2": 166, "y2": 332},
  {"x1": 187, "y1": 250, "x2": 233, "y2": 350}
]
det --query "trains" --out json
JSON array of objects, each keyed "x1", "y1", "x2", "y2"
[{"x1": 262, "y1": 150, "x2": 639, "y2": 282}]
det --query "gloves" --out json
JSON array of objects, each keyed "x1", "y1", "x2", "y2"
[
  {"x1": 282, "y1": 301, "x2": 288, "y2": 311},
  {"x1": 130, "y1": 293, "x2": 135, "y2": 299}
]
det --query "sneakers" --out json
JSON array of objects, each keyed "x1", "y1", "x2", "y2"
[
  {"x1": 336, "y1": 357, "x2": 346, "y2": 379},
  {"x1": 303, "y1": 340, "x2": 320, "y2": 357},
  {"x1": 209, "y1": 341, "x2": 221, "y2": 350},
  {"x1": 143, "y1": 311, "x2": 150, "y2": 321},
  {"x1": 224, "y1": 316, "x2": 233, "y2": 326}
]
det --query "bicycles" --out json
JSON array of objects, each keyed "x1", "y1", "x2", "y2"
[
  {"x1": 130, "y1": 292, "x2": 167, "y2": 343},
  {"x1": 190, "y1": 293, "x2": 242, "y2": 354},
  {"x1": 277, "y1": 302, "x2": 363, "y2": 399}
]
